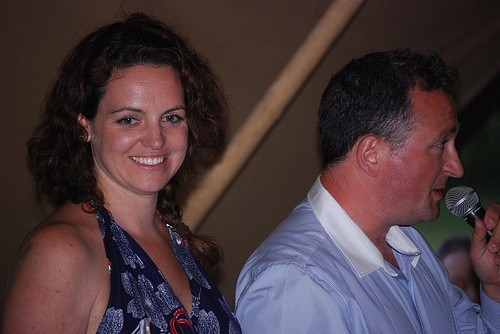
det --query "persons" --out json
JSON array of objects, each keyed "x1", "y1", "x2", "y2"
[
  {"x1": 0, "y1": 12, "x2": 243, "y2": 334},
  {"x1": 235, "y1": 49, "x2": 500, "y2": 334},
  {"x1": 435, "y1": 236, "x2": 481, "y2": 307}
]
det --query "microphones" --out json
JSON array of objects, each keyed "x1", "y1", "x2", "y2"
[{"x1": 445, "y1": 186, "x2": 493, "y2": 241}]
